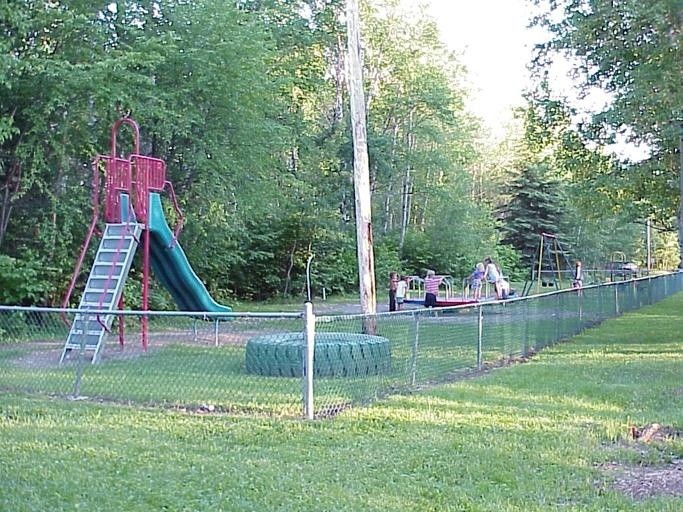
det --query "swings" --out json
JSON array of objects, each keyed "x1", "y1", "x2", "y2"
[{"x1": 540, "y1": 237, "x2": 556, "y2": 287}]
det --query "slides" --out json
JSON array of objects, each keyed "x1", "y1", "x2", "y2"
[{"x1": 120, "y1": 193, "x2": 236, "y2": 321}]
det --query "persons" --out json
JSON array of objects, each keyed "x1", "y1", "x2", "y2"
[
  {"x1": 394, "y1": 276, "x2": 411, "y2": 310},
  {"x1": 574, "y1": 261, "x2": 584, "y2": 297},
  {"x1": 465, "y1": 256, "x2": 517, "y2": 302},
  {"x1": 387, "y1": 273, "x2": 399, "y2": 312},
  {"x1": 424, "y1": 270, "x2": 444, "y2": 317}
]
